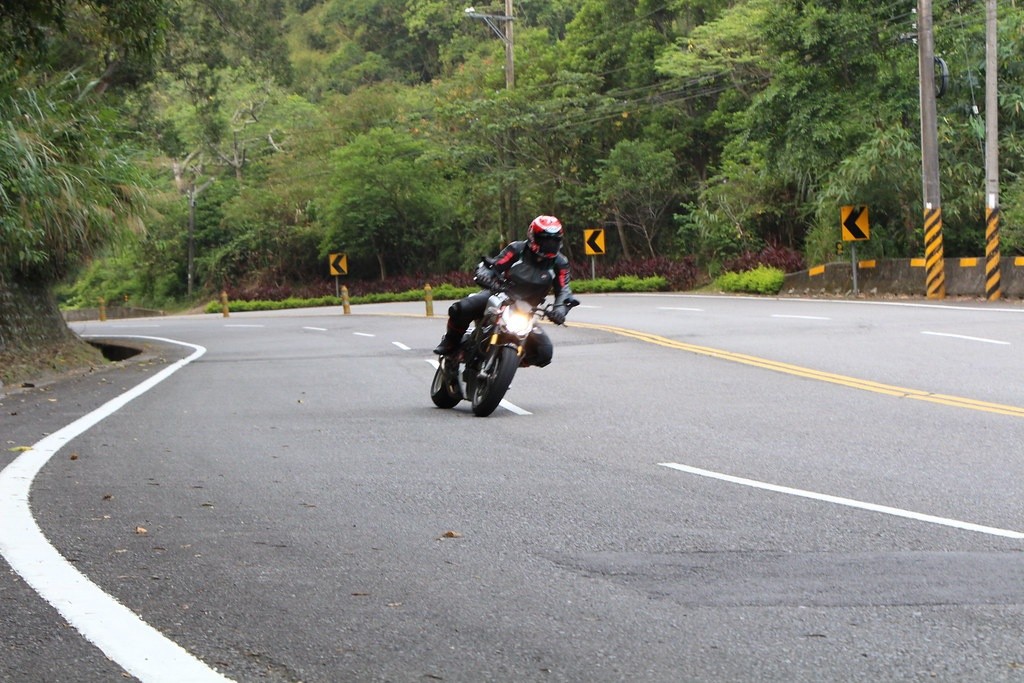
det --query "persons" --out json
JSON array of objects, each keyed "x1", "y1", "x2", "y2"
[{"x1": 432, "y1": 215, "x2": 574, "y2": 391}]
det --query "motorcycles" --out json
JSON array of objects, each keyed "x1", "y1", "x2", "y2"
[{"x1": 430, "y1": 255, "x2": 581, "y2": 416}]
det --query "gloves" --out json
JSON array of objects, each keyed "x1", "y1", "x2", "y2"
[
  {"x1": 473, "y1": 265, "x2": 498, "y2": 291},
  {"x1": 545, "y1": 305, "x2": 572, "y2": 325}
]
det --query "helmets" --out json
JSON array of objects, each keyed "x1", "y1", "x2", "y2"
[{"x1": 527, "y1": 215, "x2": 565, "y2": 258}]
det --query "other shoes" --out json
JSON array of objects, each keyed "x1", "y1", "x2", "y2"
[{"x1": 433, "y1": 332, "x2": 462, "y2": 355}]
